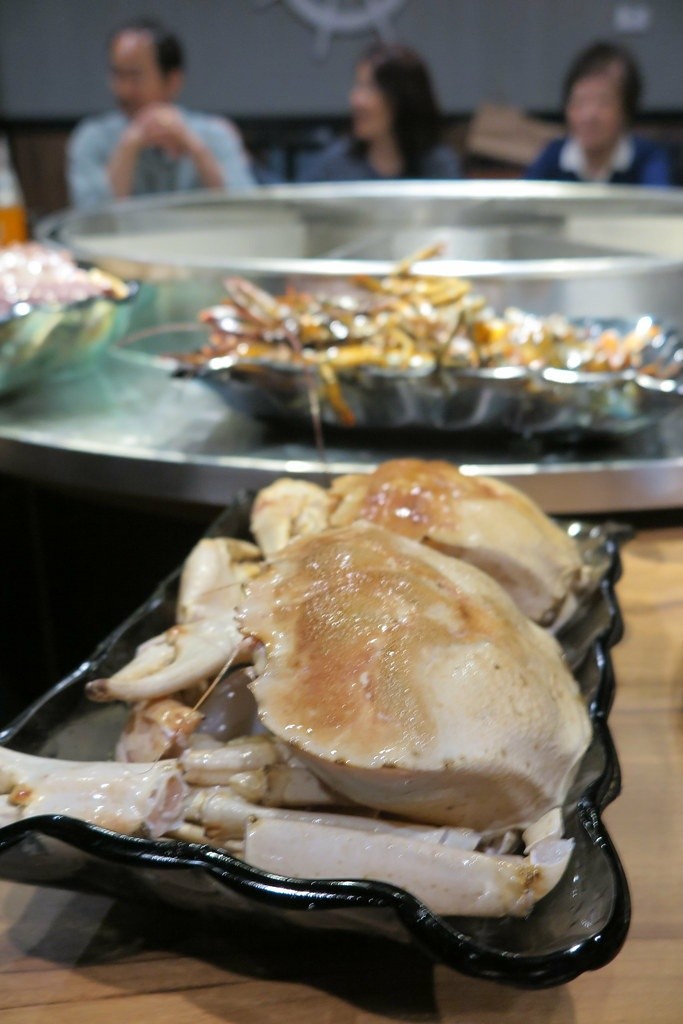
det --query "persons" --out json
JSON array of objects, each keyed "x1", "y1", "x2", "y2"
[
  {"x1": 67, "y1": 16, "x2": 257, "y2": 207},
  {"x1": 278, "y1": 43, "x2": 473, "y2": 185},
  {"x1": 516, "y1": 41, "x2": 674, "y2": 186}
]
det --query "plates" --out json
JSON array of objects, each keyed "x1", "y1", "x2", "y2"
[
  {"x1": 0, "y1": 277, "x2": 155, "y2": 398},
  {"x1": 172, "y1": 354, "x2": 683, "y2": 431},
  {"x1": 0, "y1": 488, "x2": 633, "y2": 991}
]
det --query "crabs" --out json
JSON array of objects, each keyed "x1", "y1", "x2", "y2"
[{"x1": 0, "y1": 457, "x2": 595, "y2": 920}]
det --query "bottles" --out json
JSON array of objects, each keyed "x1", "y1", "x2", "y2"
[{"x1": 0, "y1": 139, "x2": 29, "y2": 246}]
